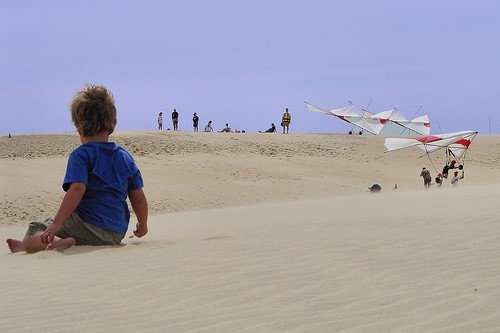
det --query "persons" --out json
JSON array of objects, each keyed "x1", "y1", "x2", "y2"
[
  {"x1": 421, "y1": 160, "x2": 464, "y2": 189},
  {"x1": 158, "y1": 112, "x2": 163, "y2": 130},
  {"x1": 172, "y1": 109, "x2": 179, "y2": 130},
  {"x1": 221, "y1": 123, "x2": 230, "y2": 133},
  {"x1": 282, "y1": 108, "x2": 292, "y2": 134},
  {"x1": 6, "y1": 81, "x2": 149, "y2": 255},
  {"x1": 259, "y1": 123, "x2": 277, "y2": 133},
  {"x1": 368, "y1": 184, "x2": 381, "y2": 193},
  {"x1": 192, "y1": 113, "x2": 199, "y2": 132},
  {"x1": 204, "y1": 121, "x2": 214, "y2": 132}
]
